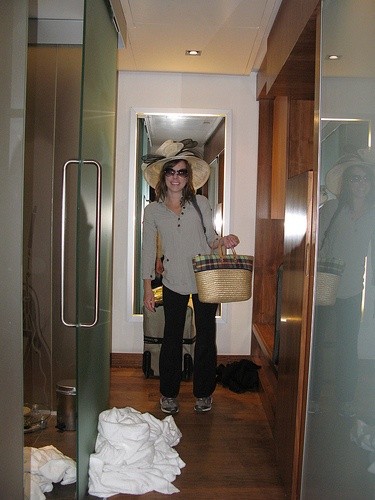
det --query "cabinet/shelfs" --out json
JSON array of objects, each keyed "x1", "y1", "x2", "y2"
[{"x1": 249, "y1": 0, "x2": 321, "y2": 500}]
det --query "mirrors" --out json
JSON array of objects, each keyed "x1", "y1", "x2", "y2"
[{"x1": 127, "y1": 106, "x2": 233, "y2": 325}]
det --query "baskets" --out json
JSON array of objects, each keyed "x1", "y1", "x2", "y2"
[
  {"x1": 191, "y1": 237, "x2": 254, "y2": 303},
  {"x1": 314, "y1": 256, "x2": 346, "y2": 306}
]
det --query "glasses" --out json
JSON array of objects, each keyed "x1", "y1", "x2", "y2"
[
  {"x1": 348, "y1": 176, "x2": 368, "y2": 183},
  {"x1": 163, "y1": 168, "x2": 188, "y2": 177}
]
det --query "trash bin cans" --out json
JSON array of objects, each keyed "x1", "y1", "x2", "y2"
[{"x1": 55, "y1": 378, "x2": 77, "y2": 431}]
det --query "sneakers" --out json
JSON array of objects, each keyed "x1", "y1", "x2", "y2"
[
  {"x1": 309, "y1": 400, "x2": 320, "y2": 414},
  {"x1": 338, "y1": 400, "x2": 356, "y2": 417},
  {"x1": 159, "y1": 395, "x2": 179, "y2": 412},
  {"x1": 194, "y1": 395, "x2": 213, "y2": 412}
]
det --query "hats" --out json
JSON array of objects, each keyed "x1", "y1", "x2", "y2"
[
  {"x1": 324, "y1": 144, "x2": 375, "y2": 196},
  {"x1": 140, "y1": 139, "x2": 210, "y2": 190}
]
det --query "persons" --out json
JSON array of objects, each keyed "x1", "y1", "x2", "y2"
[
  {"x1": 138, "y1": 138, "x2": 240, "y2": 414},
  {"x1": 305, "y1": 150, "x2": 375, "y2": 417}
]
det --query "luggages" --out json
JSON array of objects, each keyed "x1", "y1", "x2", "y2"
[{"x1": 142, "y1": 303, "x2": 195, "y2": 381}]
